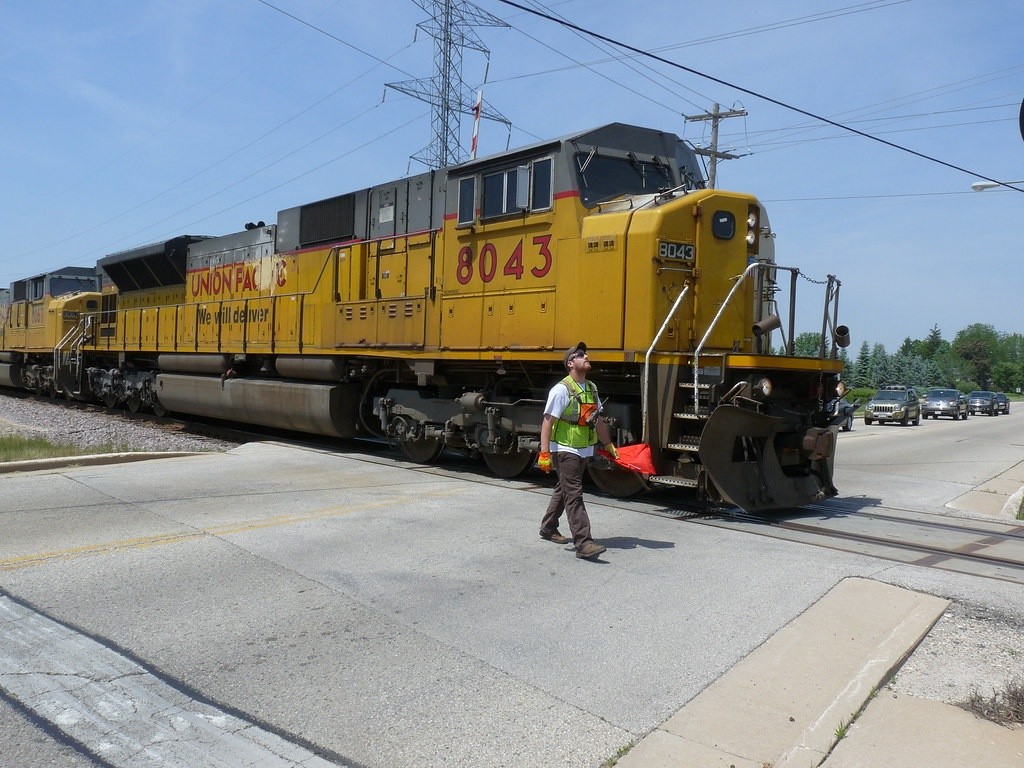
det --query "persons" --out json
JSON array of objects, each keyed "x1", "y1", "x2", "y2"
[{"x1": 538, "y1": 341, "x2": 620, "y2": 558}]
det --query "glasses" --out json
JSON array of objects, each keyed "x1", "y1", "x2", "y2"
[{"x1": 569, "y1": 352, "x2": 587, "y2": 361}]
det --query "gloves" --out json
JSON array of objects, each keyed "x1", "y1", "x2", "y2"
[
  {"x1": 604, "y1": 442, "x2": 619, "y2": 460},
  {"x1": 538, "y1": 452, "x2": 553, "y2": 472}
]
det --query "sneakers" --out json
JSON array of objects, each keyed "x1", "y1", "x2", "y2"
[
  {"x1": 539, "y1": 530, "x2": 568, "y2": 544},
  {"x1": 576, "y1": 543, "x2": 606, "y2": 558}
]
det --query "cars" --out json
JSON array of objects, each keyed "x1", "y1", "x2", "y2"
[
  {"x1": 922, "y1": 388, "x2": 970, "y2": 421},
  {"x1": 996, "y1": 393, "x2": 1011, "y2": 415},
  {"x1": 827, "y1": 396, "x2": 853, "y2": 430}
]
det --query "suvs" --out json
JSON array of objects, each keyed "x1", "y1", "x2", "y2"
[
  {"x1": 864, "y1": 384, "x2": 921, "y2": 427},
  {"x1": 968, "y1": 391, "x2": 999, "y2": 417}
]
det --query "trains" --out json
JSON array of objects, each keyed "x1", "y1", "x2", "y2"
[{"x1": 0, "y1": 121, "x2": 853, "y2": 519}]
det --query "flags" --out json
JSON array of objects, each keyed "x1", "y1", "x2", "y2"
[{"x1": 596, "y1": 443, "x2": 656, "y2": 476}]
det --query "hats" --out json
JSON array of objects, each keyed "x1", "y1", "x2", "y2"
[{"x1": 564, "y1": 342, "x2": 587, "y2": 368}]
